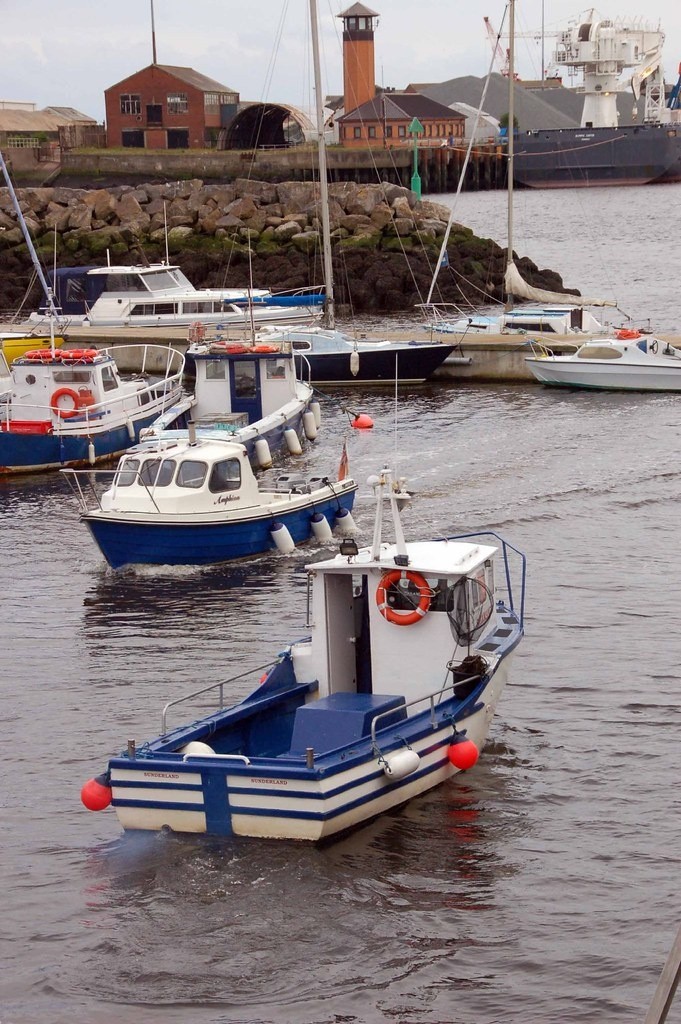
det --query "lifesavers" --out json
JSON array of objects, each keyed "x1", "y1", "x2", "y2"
[
  {"x1": 51, "y1": 388, "x2": 79, "y2": 418},
  {"x1": 376, "y1": 569, "x2": 431, "y2": 626},
  {"x1": 188, "y1": 321, "x2": 204, "y2": 342},
  {"x1": 61, "y1": 348, "x2": 100, "y2": 363},
  {"x1": 613, "y1": 330, "x2": 641, "y2": 340},
  {"x1": 249, "y1": 345, "x2": 279, "y2": 352},
  {"x1": 209, "y1": 341, "x2": 248, "y2": 353},
  {"x1": 25, "y1": 349, "x2": 63, "y2": 362}
]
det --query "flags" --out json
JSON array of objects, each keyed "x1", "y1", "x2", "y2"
[{"x1": 339, "y1": 445, "x2": 349, "y2": 481}]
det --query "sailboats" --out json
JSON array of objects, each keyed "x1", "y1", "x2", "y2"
[
  {"x1": 182, "y1": 0, "x2": 472, "y2": 390},
  {"x1": 413, "y1": 0, "x2": 617, "y2": 336}
]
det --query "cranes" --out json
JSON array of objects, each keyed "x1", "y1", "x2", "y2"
[
  {"x1": 486, "y1": 8, "x2": 594, "y2": 39},
  {"x1": 483, "y1": 16, "x2": 521, "y2": 81}
]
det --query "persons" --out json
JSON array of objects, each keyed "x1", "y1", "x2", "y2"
[{"x1": 447, "y1": 132, "x2": 453, "y2": 146}]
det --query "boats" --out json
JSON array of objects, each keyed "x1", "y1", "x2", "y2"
[
  {"x1": 0, "y1": 344, "x2": 185, "y2": 474},
  {"x1": 525, "y1": 331, "x2": 681, "y2": 391},
  {"x1": 108, "y1": 469, "x2": 525, "y2": 841},
  {"x1": 59, "y1": 419, "x2": 359, "y2": 571},
  {"x1": 513, "y1": 19, "x2": 681, "y2": 189},
  {"x1": 27, "y1": 264, "x2": 326, "y2": 327},
  {"x1": 0, "y1": 314, "x2": 71, "y2": 375},
  {"x1": 138, "y1": 322, "x2": 318, "y2": 491}
]
